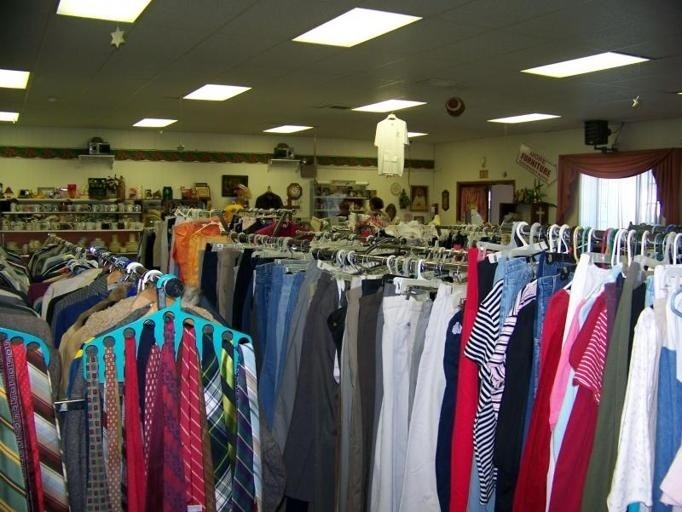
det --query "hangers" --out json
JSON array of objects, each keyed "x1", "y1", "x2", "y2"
[
  {"x1": 156, "y1": 201, "x2": 680, "y2": 297},
  {"x1": 386, "y1": 111, "x2": 398, "y2": 120},
  {"x1": 0, "y1": 243, "x2": 29, "y2": 308},
  {"x1": 29, "y1": 230, "x2": 186, "y2": 324}
]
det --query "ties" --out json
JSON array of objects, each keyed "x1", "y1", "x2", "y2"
[
  {"x1": 64, "y1": 315, "x2": 264, "y2": 512},
  {"x1": 0, "y1": 339, "x2": 66, "y2": 512}
]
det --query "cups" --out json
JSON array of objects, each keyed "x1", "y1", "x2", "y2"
[
  {"x1": 0, "y1": 203, "x2": 144, "y2": 231},
  {"x1": 68, "y1": 183, "x2": 76, "y2": 199}
]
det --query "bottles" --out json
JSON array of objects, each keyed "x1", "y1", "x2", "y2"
[
  {"x1": 6, "y1": 240, "x2": 41, "y2": 255},
  {"x1": 181, "y1": 188, "x2": 196, "y2": 200},
  {"x1": 117, "y1": 175, "x2": 126, "y2": 202},
  {"x1": 163, "y1": 186, "x2": 172, "y2": 200},
  {"x1": 89, "y1": 233, "x2": 139, "y2": 254}
]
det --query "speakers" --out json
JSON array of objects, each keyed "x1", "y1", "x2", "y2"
[{"x1": 583, "y1": 119, "x2": 609, "y2": 146}]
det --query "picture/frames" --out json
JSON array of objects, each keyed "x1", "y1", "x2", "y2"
[
  {"x1": 409, "y1": 184, "x2": 430, "y2": 213},
  {"x1": 220, "y1": 174, "x2": 249, "y2": 198}
]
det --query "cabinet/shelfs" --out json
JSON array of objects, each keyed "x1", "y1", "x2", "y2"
[
  {"x1": 1, "y1": 196, "x2": 146, "y2": 265},
  {"x1": 133, "y1": 195, "x2": 213, "y2": 230},
  {"x1": 308, "y1": 180, "x2": 371, "y2": 223}
]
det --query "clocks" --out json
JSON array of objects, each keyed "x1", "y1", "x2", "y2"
[{"x1": 287, "y1": 182, "x2": 303, "y2": 200}]
